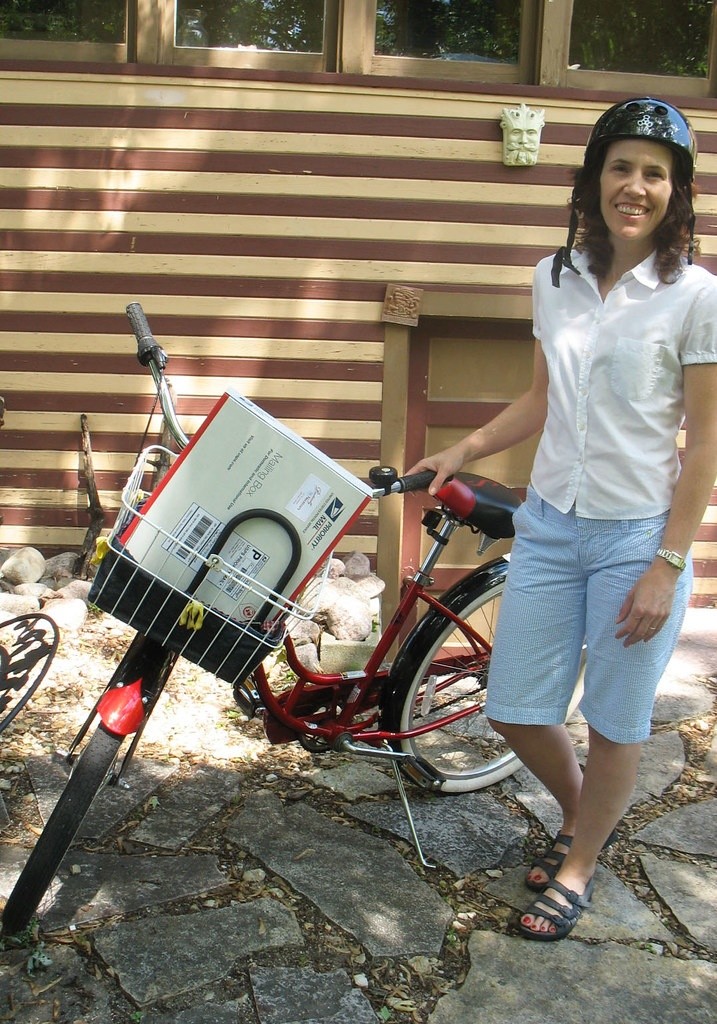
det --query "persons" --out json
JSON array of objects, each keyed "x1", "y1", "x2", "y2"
[{"x1": 405, "y1": 94, "x2": 717, "y2": 939}]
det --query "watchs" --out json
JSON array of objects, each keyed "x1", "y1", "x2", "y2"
[{"x1": 655, "y1": 548, "x2": 686, "y2": 572}]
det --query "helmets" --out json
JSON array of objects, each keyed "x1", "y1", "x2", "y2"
[{"x1": 587, "y1": 96, "x2": 698, "y2": 182}]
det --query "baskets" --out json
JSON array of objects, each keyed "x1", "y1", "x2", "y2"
[{"x1": 89, "y1": 444, "x2": 335, "y2": 690}]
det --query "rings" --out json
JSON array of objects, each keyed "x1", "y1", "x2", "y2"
[{"x1": 648, "y1": 627, "x2": 656, "y2": 630}]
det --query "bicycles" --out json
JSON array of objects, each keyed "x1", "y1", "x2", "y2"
[{"x1": 1, "y1": 301, "x2": 587, "y2": 935}]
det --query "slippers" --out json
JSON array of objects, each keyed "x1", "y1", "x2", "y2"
[
  {"x1": 525, "y1": 823, "x2": 618, "y2": 892},
  {"x1": 518, "y1": 874, "x2": 593, "y2": 939}
]
